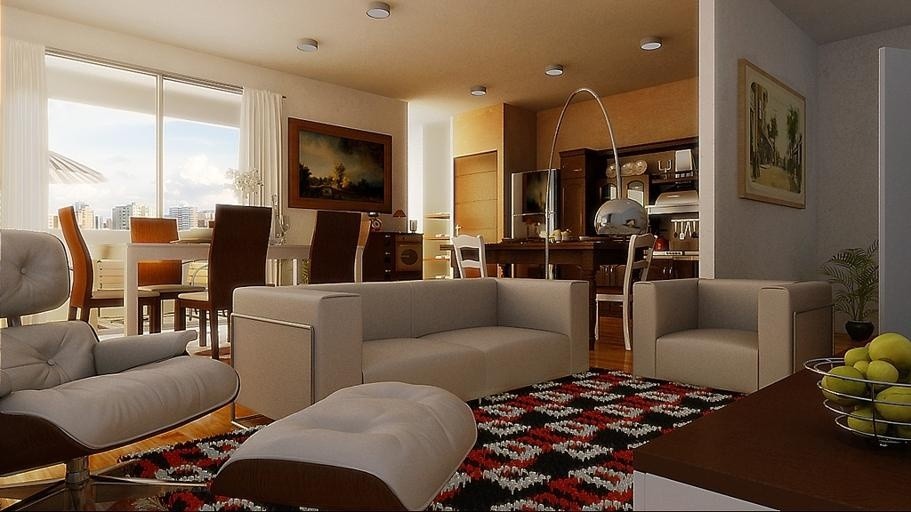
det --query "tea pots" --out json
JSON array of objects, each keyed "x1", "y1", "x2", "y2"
[{"x1": 655, "y1": 234, "x2": 669, "y2": 250}]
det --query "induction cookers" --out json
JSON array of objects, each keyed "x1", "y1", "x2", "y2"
[{"x1": 652, "y1": 250, "x2": 699, "y2": 256}]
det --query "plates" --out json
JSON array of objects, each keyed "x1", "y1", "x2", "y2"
[
  {"x1": 620, "y1": 162, "x2": 634, "y2": 176},
  {"x1": 633, "y1": 160, "x2": 647, "y2": 175},
  {"x1": 605, "y1": 163, "x2": 617, "y2": 178}
]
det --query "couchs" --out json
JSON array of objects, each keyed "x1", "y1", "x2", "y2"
[{"x1": 229, "y1": 278, "x2": 589, "y2": 430}]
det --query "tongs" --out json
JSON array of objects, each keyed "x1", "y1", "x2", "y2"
[{"x1": 684, "y1": 222, "x2": 693, "y2": 237}]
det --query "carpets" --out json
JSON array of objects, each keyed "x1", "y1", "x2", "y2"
[{"x1": 117, "y1": 367, "x2": 746, "y2": 512}]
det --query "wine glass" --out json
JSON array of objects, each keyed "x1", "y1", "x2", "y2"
[{"x1": 279, "y1": 215, "x2": 290, "y2": 245}]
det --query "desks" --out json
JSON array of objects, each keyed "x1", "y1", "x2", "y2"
[
  {"x1": 633, "y1": 345, "x2": 910, "y2": 511},
  {"x1": 439, "y1": 240, "x2": 644, "y2": 350}
]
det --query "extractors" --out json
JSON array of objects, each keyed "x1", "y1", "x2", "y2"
[{"x1": 644, "y1": 191, "x2": 699, "y2": 215}]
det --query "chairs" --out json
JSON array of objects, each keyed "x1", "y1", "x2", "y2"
[
  {"x1": 633, "y1": 278, "x2": 833, "y2": 394},
  {"x1": 451, "y1": 235, "x2": 489, "y2": 279},
  {"x1": 90, "y1": 260, "x2": 164, "y2": 330},
  {"x1": 594, "y1": 234, "x2": 659, "y2": 351},
  {"x1": 178, "y1": 259, "x2": 228, "y2": 321},
  {"x1": 0, "y1": 230, "x2": 240, "y2": 511}
]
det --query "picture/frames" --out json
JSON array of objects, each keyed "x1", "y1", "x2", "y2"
[
  {"x1": 738, "y1": 58, "x2": 807, "y2": 209},
  {"x1": 288, "y1": 117, "x2": 392, "y2": 216}
]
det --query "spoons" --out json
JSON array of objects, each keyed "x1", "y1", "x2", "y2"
[{"x1": 674, "y1": 221, "x2": 678, "y2": 237}]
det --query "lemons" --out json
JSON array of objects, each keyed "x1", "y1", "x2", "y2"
[{"x1": 821, "y1": 333, "x2": 911, "y2": 438}]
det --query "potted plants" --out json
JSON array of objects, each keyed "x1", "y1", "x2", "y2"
[{"x1": 820, "y1": 240, "x2": 878, "y2": 340}]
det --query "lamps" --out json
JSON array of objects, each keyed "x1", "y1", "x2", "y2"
[
  {"x1": 393, "y1": 209, "x2": 407, "y2": 233},
  {"x1": 545, "y1": 88, "x2": 648, "y2": 279}
]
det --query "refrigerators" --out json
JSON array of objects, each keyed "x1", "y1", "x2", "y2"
[{"x1": 511, "y1": 169, "x2": 561, "y2": 278}]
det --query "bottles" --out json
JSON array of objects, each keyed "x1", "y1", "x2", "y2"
[{"x1": 269, "y1": 193, "x2": 285, "y2": 247}]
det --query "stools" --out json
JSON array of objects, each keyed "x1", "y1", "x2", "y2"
[{"x1": 209, "y1": 380, "x2": 478, "y2": 512}]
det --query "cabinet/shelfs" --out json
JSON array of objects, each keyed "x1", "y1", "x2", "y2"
[
  {"x1": 363, "y1": 232, "x2": 424, "y2": 282},
  {"x1": 423, "y1": 213, "x2": 452, "y2": 279},
  {"x1": 557, "y1": 136, "x2": 699, "y2": 318}
]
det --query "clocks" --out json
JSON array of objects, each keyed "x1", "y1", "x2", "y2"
[{"x1": 371, "y1": 218, "x2": 383, "y2": 231}]
